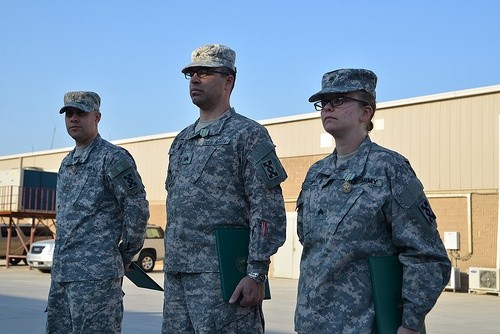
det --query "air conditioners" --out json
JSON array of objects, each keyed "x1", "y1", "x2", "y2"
[
  {"x1": 445, "y1": 267, "x2": 461, "y2": 290},
  {"x1": 469, "y1": 267, "x2": 497, "y2": 291}
]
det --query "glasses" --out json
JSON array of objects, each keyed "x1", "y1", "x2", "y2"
[
  {"x1": 184, "y1": 69, "x2": 228, "y2": 79},
  {"x1": 314, "y1": 96, "x2": 369, "y2": 111}
]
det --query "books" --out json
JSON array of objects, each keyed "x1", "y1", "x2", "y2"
[
  {"x1": 124, "y1": 260, "x2": 165, "y2": 292},
  {"x1": 368, "y1": 256, "x2": 427, "y2": 334},
  {"x1": 214, "y1": 228, "x2": 272, "y2": 304}
]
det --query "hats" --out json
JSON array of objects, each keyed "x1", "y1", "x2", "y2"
[
  {"x1": 59, "y1": 91, "x2": 101, "y2": 112},
  {"x1": 182, "y1": 44, "x2": 237, "y2": 74},
  {"x1": 309, "y1": 69, "x2": 378, "y2": 102}
]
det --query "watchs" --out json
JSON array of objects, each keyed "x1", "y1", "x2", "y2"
[{"x1": 246, "y1": 271, "x2": 268, "y2": 285}]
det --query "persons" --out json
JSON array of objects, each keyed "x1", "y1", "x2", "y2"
[
  {"x1": 161, "y1": 43, "x2": 288, "y2": 334},
  {"x1": 294, "y1": 68, "x2": 452, "y2": 334},
  {"x1": 45, "y1": 91, "x2": 150, "y2": 334}
]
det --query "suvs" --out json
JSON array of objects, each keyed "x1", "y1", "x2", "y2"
[{"x1": 26, "y1": 223, "x2": 166, "y2": 274}]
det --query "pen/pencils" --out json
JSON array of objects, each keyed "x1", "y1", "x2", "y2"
[{"x1": 255, "y1": 219, "x2": 268, "y2": 235}]
